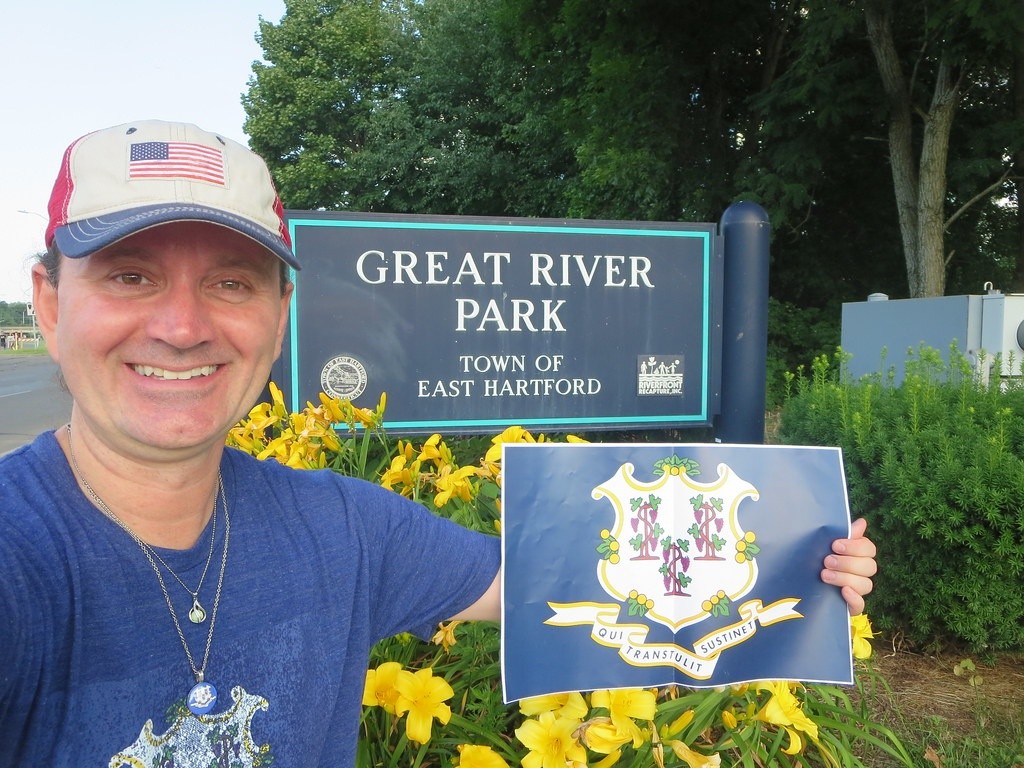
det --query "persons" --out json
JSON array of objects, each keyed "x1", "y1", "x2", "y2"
[{"x1": 0, "y1": 119, "x2": 878, "y2": 768}]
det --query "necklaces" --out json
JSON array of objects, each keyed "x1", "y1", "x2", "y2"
[
  {"x1": 66, "y1": 423, "x2": 219, "y2": 624},
  {"x1": 67, "y1": 425, "x2": 229, "y2": 713}
]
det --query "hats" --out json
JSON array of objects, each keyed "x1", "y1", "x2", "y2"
[{"x1": 45, "y1": 120, "x2": 304, "y2": 272}]
